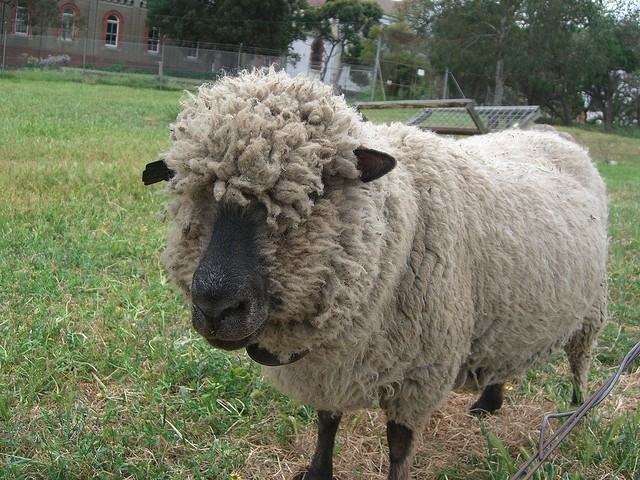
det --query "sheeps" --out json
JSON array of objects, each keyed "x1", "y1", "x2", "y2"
[{"x1": 141, "y1": 61, "x2": 611, "y2": 480}]
